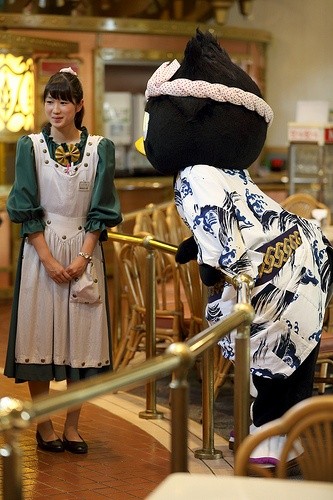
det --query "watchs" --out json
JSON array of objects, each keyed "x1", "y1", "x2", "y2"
[{"x1": 78, "y1": 252, "x2": 93, "y2": 263}]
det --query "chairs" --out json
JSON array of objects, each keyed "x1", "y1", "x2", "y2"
[{"x1": 109, "y1": 193, "x2": 333, "y2": 480}]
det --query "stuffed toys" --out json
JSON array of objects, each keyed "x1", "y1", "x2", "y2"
[{"x1": 135, "y1": 28, "x2": 333, "y2": 474}]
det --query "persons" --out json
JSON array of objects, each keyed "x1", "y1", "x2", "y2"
[{"x1": 3, "y1": 67, "x2": 123, "y2": 455}]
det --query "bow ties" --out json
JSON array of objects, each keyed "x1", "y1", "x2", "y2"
[{"x1": 55, "y1": 143, "x2": 80, "y2": 167}]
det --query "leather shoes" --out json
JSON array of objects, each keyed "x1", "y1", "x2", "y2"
[
  {"x1": 62, "y1": 432, "x2": 88, "y2": 454},
  {"x1": 36, "y1": 419, "x2": 64, "y2": 451}
]
